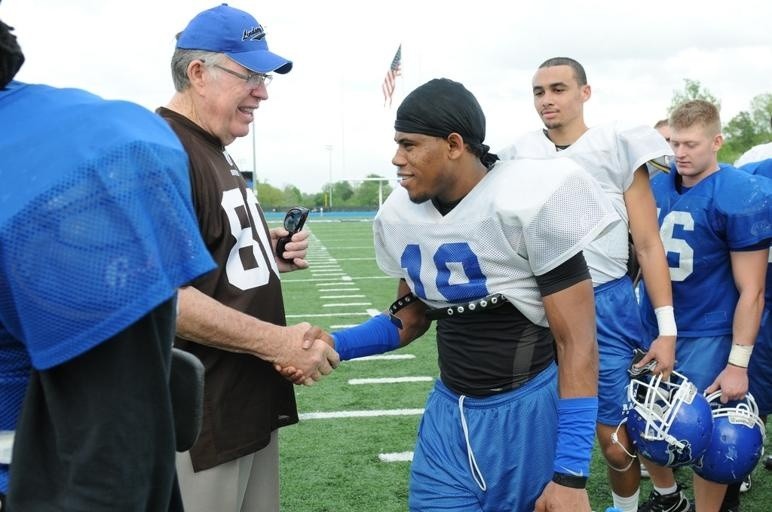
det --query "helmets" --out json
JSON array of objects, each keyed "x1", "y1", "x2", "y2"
[
  {"x1": 610, "y1": 349, "x2": 713, "y2": 467},
  {"x1": 691, "y1": 385, "x2": 767, "y2": 484}
]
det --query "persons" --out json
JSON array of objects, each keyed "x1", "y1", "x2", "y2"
[
  {"x1": 732, "y1": 158, "x2": 772, "y2": 492},
  {"x1": 147, "y1": 3, "x2": 341, "y2": 511},
  {"x1": 272, "y1": 76, "x2": 603, "y2": 512},
  {"x1": 500, "y1": 56, "x2": 681, "y2": 511},
  {"x1": 654, "y1": 119, "x2": 671, "y2": 145},
  {"x1": 0, "y1": 15, "x2": 218, "y2": 512},
  {"x1": 640, "y1": 100, "x2": 772, "y2": 512}
]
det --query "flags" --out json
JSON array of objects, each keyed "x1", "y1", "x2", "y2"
[{"x1": 381, "y1": 43, "x2": 402, "y2": 109}]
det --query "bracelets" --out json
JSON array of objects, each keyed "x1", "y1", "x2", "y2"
[
  {"x1": 652, "y1": 305, "x2": 679, "y2": 338},
  {"x1": 727, "y1": 343, "x2": 754, "y2": 369},
  {"x1": 556, "y1": 396, "x2": 598, "y2": 489},
  {"x1": 550, "y1": 472, "x2": 587, "y2": 488},
  {"x1": 326, "y1": 314, "x2": 400, "y2": 361}
]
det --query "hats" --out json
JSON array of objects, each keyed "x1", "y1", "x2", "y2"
[{"x1": 176, "y1": 3, "x2": 293, "y2": 76}]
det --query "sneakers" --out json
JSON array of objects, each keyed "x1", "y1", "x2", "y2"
[
  {"x1": 738, "y1": 474, "x2": 752, "y2": 491},
  {"x1": 637, "y1": 481, "x2": 692, "y2": 512}
]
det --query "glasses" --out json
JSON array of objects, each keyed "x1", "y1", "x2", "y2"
[
  {"x1": 211, "y1": 64, "x2": 274, "y2": 87},
  {"x1": 275, "y1": 205, "x2": 310, "y2": 264}
]
